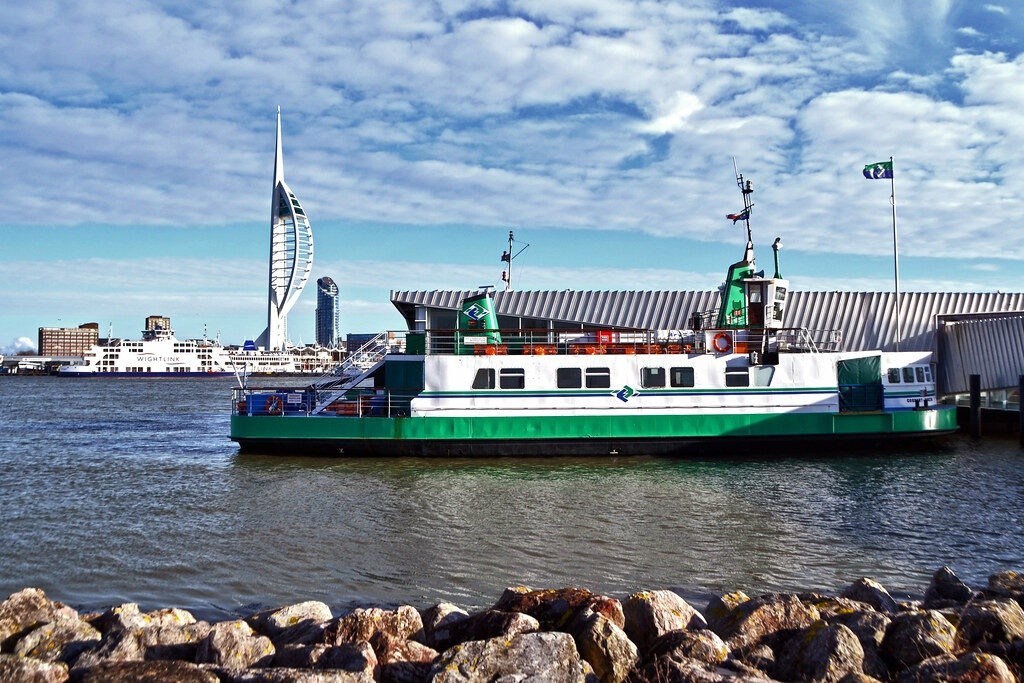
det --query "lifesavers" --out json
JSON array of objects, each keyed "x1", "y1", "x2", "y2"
[
  {"x1": 265, "y1": 396, "x2": 282, "y2": 414},
  {"x1": 713, "y1": 332, "x2": 732, "y2": 352}
]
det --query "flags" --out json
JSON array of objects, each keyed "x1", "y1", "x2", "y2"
[{"x1": 863, "y1": 162, "x2": 893, "y2": 179}]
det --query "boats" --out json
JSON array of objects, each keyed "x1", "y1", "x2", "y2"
[
  {"x1": 56, "y1": 322, "x2": 252, "y2": 377},
  {"x1": 226, "y1": 153, "x2": 977, "y2": 457}
]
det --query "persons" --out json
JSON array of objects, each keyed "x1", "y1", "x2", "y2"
[
  {"x1": 920, "y1": 387, "x2": 927, "y2": 397},
  {"x1": 362, "y1": 390, "x2": 385, "y2": 417}
]
